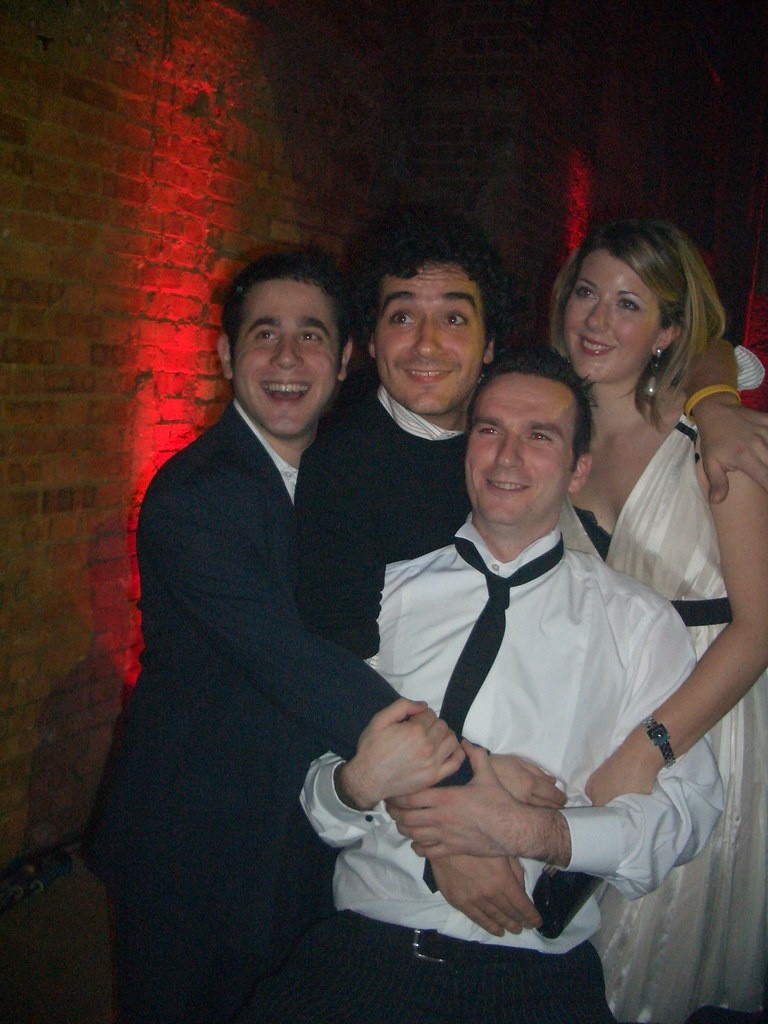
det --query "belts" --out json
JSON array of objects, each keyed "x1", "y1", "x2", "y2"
[{"x1": 333, "y1": 909, "x2": 538, "y2": 970}]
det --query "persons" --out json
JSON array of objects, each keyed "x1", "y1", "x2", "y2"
[
  {"x1": 549, "y1": 211, "x2": 768, "y2": 1024},
  {"x1": 290, "y1": 210, "x2": 768, "y2": 935},
  {"x1": 81, "y1": 237, "x2": 569, "y2": 1024},
  {"x1": 296, "y1": 347, "x2": 723, "y2": 1023}
]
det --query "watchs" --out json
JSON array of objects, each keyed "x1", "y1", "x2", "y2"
[{"x1": 641, "y1": 717, "x2": 677, "y2": 768}]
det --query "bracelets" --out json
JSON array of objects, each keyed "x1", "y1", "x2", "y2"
[{"x1": 684, "y1": 386, "x2": 741, "y2": 421}]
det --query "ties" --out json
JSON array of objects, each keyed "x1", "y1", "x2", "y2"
[{"x1": 424, "y1": 534, "x2": 565, "y2": 893}]
